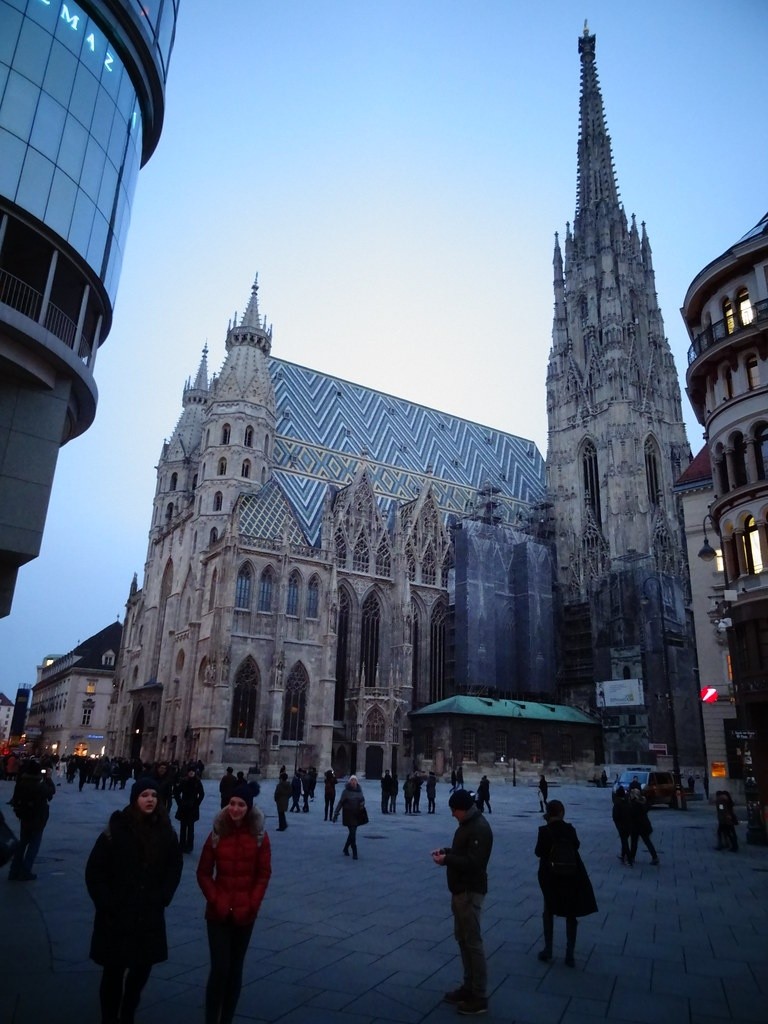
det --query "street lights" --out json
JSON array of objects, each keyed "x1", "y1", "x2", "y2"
[
  {"x1": 640, "y1": 576, "x2": 687, "y2": 811},
  {"x1": 697, "y1": 513, "x2": 767, "y2": 846}
]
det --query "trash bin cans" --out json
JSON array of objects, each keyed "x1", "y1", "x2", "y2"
[{"x1": 248, "y1": 767, "x2": 260, "y2": 781}]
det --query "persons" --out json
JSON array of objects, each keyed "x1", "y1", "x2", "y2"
[
  {"x1": 0, "y1": 737, "x2": 337, "y2": 852},
  {"x1": 8, "y1": 761, "x2": 56, "y2": 881},
  {"x1": 450, "y1": 766, "x2": 464, "y2": 794},
  {"x1": 688, "y1": 775, "x2": 695, "y2": 792},
  {"x1": 86, "y1": 779, "x2": 185, "y2": 1023},
  {"x1": 713, "y1": 790, "x2": 739, "y2": 852},
  {"x1": 332, "y1": 775, "x2": 370, "y2": 859},
  {"x1": 628, "y1": 776, "x2": 641, "y2": 790},
  {"x1": 537, "y1": 775, "x2": 549, "y2": 812},
  {"x1": 534, "y1": 799, "x2": 599, "y2": 966},
  {"x1": 612, "y1": 786, "x2": 660, "y2": 866},
  {"x1": 429, "y1": 789, "x2": 494, "y2": 1014},
  {"x1": 376, "y1": 769, "x2": 492, "y2": 815},
  {"x1": 197, "y1": 782, "x2": 273, "y2": 1022},
  {"x1": 593, "y1": 772, "x2": 618, "y2": 786}
]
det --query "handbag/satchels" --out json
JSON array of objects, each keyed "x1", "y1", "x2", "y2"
[{"x1": 358, "y1": 803, "x2": 369, "y2": 825}]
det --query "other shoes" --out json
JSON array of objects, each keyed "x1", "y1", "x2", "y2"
[
  {"x1": 443, "y1": 990, "x2": 465, "y2": 1004},
  {"x1": 7, "y1": 871, "x2": 20, "y2": 881},
  {"x1": 17, "y1": 870, "x2": 38, "y2": 881},
  {"x1": 456, "y1": 998, "x2": 488, "y2": 1014}
]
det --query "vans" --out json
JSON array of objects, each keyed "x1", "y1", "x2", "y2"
[{"x1": 611, "y1": 769, "x2": 677, "y2": 810}]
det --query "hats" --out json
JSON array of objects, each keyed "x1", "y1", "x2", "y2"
[
  {"x1": 227, "y1": 781, "x2": 261, "y2": 811},
  {"x1": 448, "y1": 789, "x2": 475, "y2": 811},
  {"x1": 348, "y1": 775, "x2": 359, "y2": 784},
  {"x1": 129, "y1": 778, "x2": 162, "y2": 806},
  {"x1": 226, "y1": 767, "x2": 234, "y2": 773}
]
